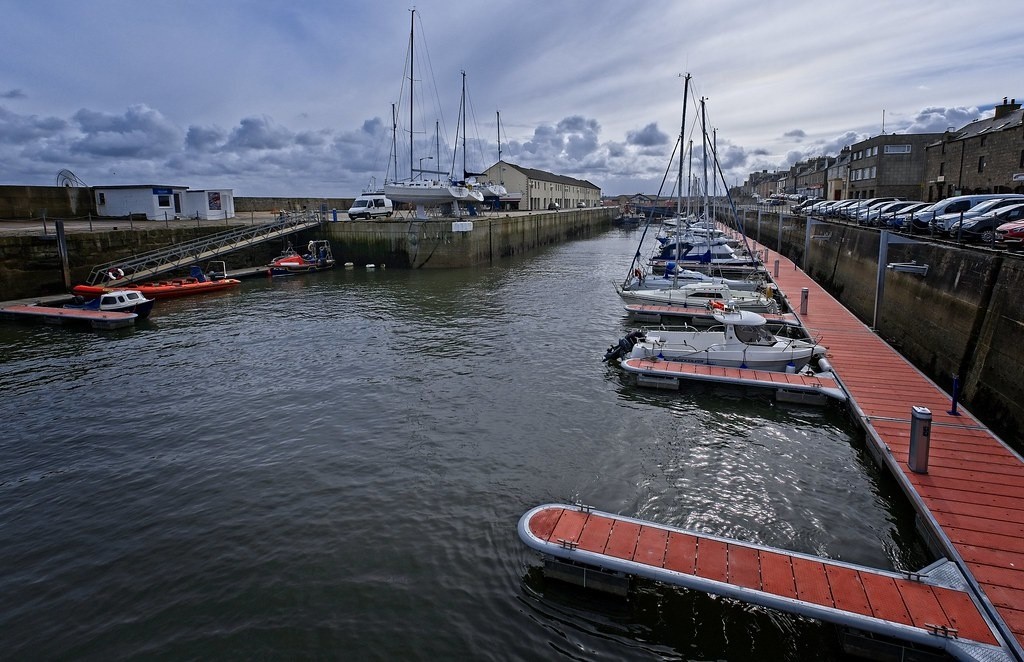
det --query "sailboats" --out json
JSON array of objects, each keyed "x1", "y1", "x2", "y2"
[
  {"x1": 602, "y1": 71, "x2": 829, "y2": 372},
  {"x1": 382, "y1": 5, "x2": 508, "y2": 203}
]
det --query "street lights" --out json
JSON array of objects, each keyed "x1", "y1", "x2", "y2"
[{"x1": 420, "y1": 156, "x2": 433, "y2": 180}]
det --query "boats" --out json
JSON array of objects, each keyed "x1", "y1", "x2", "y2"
[
  {"x1": 70, "y1": 265, "x2": 241, "y2": 298},
  {"x1": 62, "y1": 290, "x2": 156, "y2": 322},
  {"x1": 268, "y1": 239, "x2": 336, "y2": 271}
]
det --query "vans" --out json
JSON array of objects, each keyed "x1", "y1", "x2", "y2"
[{"x1": 348, "y1": 195, "x2": 394, "y2": 221}]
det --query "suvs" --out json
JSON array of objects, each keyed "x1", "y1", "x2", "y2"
[
  {"x1": 548, "y1": 202, "x2": 561, "y2": 210},
  {"x1": 577, "y1": 202, "x2": 587, "y2": 208}
]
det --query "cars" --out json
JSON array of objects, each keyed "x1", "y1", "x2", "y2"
[
  {"x1": 909, "y1": 194, "x2": 1024, "y2": 253},
  {"x1": 600, "y1": 201, "x2": 604, "y2": 206},
  {"x1": 790, "y1": 200, "x2": 840, "y2": 216},
  {"x1": 826, "y1": 199, "x2": 868, "y2": 220},
  {"x1": 847, "y1": 197, "x2": 936, "y2": 234},
  {"x1": 750, "y1": 193, "x2": 804, "y2": 207}
]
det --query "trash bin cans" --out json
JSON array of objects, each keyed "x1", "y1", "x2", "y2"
[{"x1": 594, "y1": 203, "x2": 596, "y2": 207}]
